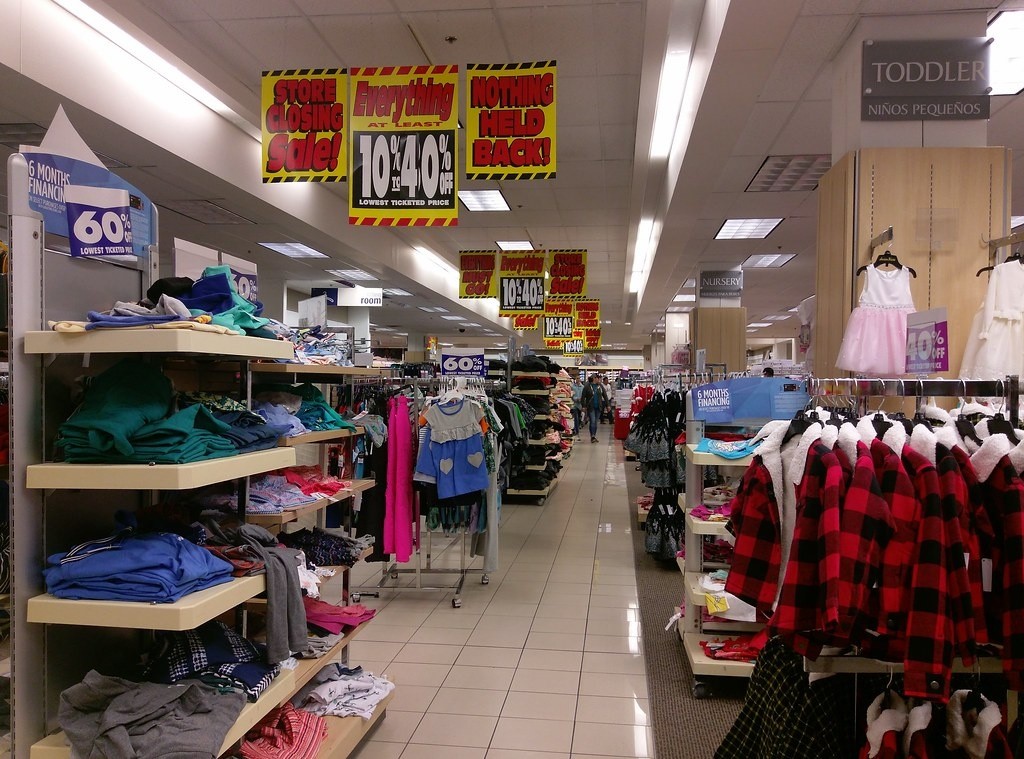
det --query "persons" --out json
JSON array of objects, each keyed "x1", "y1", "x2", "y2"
[
  {"x1": 581, "y1": 373, "x2": 615, "y2": 443},
  {"x1": 571, "y1": 374, "x2": 586, "y2": 441}
]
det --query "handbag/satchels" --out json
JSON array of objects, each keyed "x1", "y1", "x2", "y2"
[{"x1": 580, "y1": 406, "x2": 587, "y2": 418}]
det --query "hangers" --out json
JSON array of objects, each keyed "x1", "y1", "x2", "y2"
[
  {"x1": 856, "y1": 230, "x2": 917, "y2": 278},
  {"x1": 334, "y1": 362, "x2": 514, "y2": 414},
  {"x1": 976, "y1": 231, "x2": 1024, "y2": 277},
  {"x1": 631, "y1": 368, "x2": 1024, "y2": 447},
  {"x1": 877, "y1": 654, "x2": 988, "y2": 724}
]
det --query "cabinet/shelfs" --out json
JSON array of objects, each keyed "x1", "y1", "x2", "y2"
[
  {"x1": 487, "y1": 366, "x2": 639, "y2": 506},
  {"x1": 5, "y1": 327, "x2": 295, "y2": 759},
  {"x1": 236, "y1": 359, "x2": 395, "y2": 758},
  {"x1": 636, "y1": 443, "x2": 767, "y2": 699}
]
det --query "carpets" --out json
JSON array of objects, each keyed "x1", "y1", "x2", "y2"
[{"x1": 622, "y1": 461, "x2": 747, "y2": 759}]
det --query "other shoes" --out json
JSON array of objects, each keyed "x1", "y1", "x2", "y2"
[
  {"x1": 609, "y1": 420, "x2": 614, "y2": 424},
  {"x1": 600, "y1": 421, "x2": 606, "y2": 424},
  {"x1": 591, "y1": 437, "x2": 596, "y2": 443},
  {"x1": 595, "y1": 439, "x2": 599, "y2": 442},
  {"x1": 576, "y1": 435, "x2": 581, "y2": 441}
]
